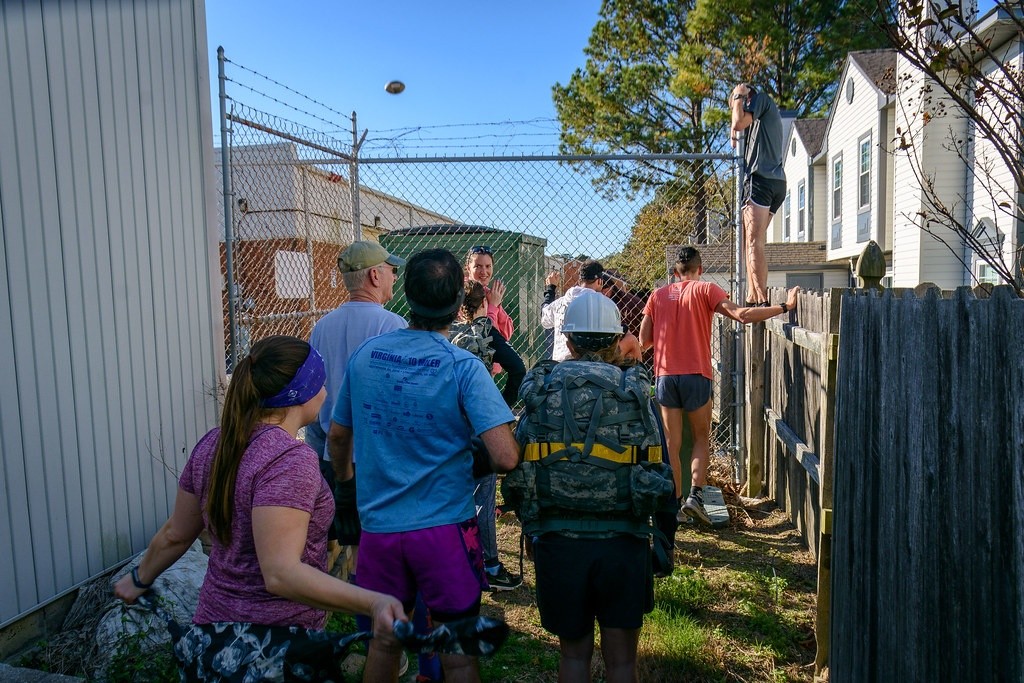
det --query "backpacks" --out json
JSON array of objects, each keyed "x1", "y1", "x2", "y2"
[{"x1": 449, "y1": 316, "x2": 497, "y2": 374}]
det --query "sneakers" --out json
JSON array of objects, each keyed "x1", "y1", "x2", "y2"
[
  {"x1": 681, "y1": 498, "x2": 712, "y2": 526},
  {"x1": 676, "y1": 498, "x2": 687, "y2": 522},
  {"x1": 482, "y1": 563, "x2": 523, "y2": 590}
]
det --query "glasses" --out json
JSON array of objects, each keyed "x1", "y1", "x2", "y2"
[
  {"x1": 467, "y1": 246, "x2": 492, "y2": 265},
  {"x1": 375, "y1": 266, "x2": 397, "y2": 274}
]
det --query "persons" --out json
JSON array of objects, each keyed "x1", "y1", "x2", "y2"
[
  {"x1": 598, "y1": 273, "x2": 654, "y2": 387},
  {"x1": 542, "y1": 259, "x2": 643, "y2": 364},
  {"x1": 728, "y1": 84, "x2": 787, "y2": 308},
  {"x1": 501, "y1": 291, "x2": 679, "y2": 683},
  {"x1": 326, "y1": 249, "x2": 521, "y2": 683},
  {"x1": 305, "y1": 241, "x2": 410, "y2": 675},
  {"x1": 465, "y1": 245, "x2": 513, "y2": 379},
  {"x1": 639, "y1": 247, "x2": 802, "y2": 527},
  {"x1": 113, "y1": 334, "x2": 409, "y2": 654},
  {"x1": 446, "y1": 280, "x2": 527, "y2": 592}
]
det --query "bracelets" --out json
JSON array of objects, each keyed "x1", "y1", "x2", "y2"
[{"x1": 780, "y1": 303, "x2": 788, "y2": 313}]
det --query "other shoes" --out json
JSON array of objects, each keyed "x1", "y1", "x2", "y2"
[{"x1": 399, "y1": 651, "x2": 408, "y2": 677}]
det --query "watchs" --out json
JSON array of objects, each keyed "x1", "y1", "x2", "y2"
[
  {"x1": 133, "y1": 566, "x2": 154, "y2": 589},
  {"x1": 733, "y1": 94, "x2": 744, "y2": 100}
]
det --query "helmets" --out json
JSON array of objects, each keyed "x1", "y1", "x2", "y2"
[{"x1": 560, "y1": 293, "x2": 625, "y2": 335}]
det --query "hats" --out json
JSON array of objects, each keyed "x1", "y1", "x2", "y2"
[{"x1": 337, "y1": 241, "x2": 406, "y2": 273}]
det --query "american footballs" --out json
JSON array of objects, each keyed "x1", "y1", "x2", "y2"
[{"x1": 384, "y1": 80, "x2": 406, "y2": 95}]
